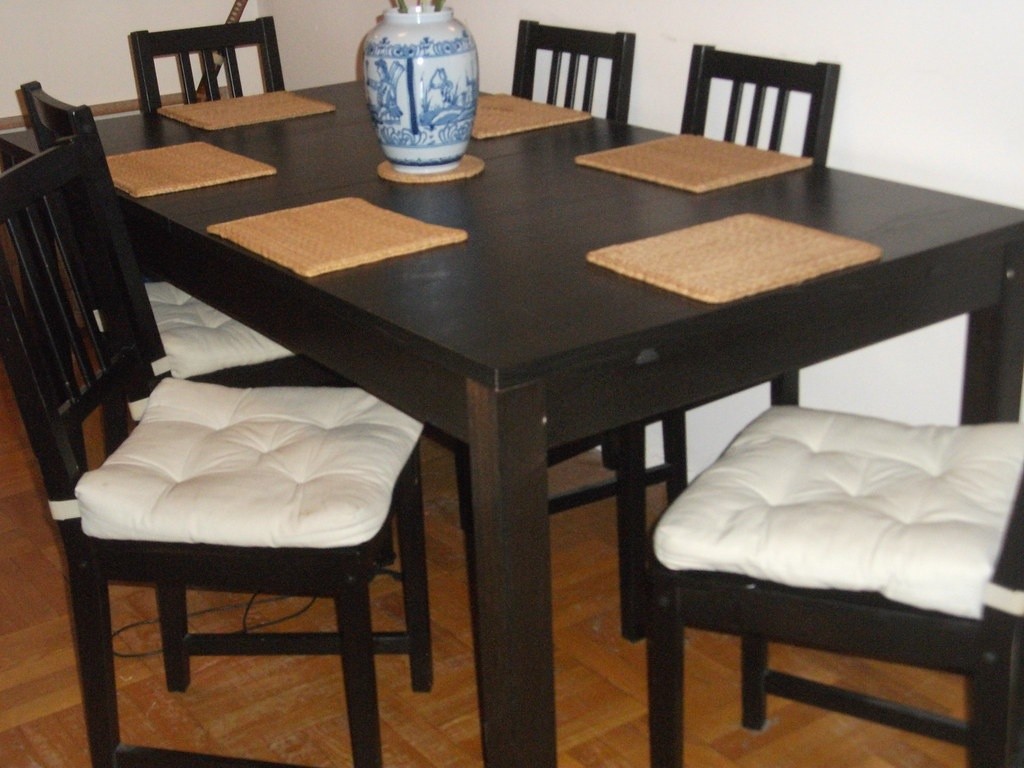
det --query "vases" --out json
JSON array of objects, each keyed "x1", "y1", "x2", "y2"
[{"x1": 362, "y1": 6, "x2": 480, "y2": 175}]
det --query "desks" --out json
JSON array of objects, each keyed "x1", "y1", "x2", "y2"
[{"x1": 2, "y1": 77, "x2": 1023, "y2": 768}]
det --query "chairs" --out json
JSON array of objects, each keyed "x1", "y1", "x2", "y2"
[
  {"x1": 451, "y1": 42, "x2": 842, "y2": 643},
  {"x1": 508, "y1": 18, "x2": 635, "y2": 122},
  {"x1": 128, "y1": 16, "x2": 288, "y2": 116},
  {"x1": 18, "y1": 79, "x2": 363, "y2": 391},
  {"x1": 630, "y1": 399, "x2": 1023, "y2": 768},
  {"x1": 0, "y1": 133, "x2": 437, "y2": 768}
]
